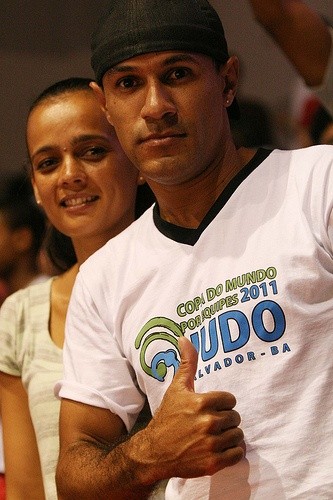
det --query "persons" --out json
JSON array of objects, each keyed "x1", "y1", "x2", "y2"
[
  {"x1": 55, "y1": 0, "x2": 333, "y2": 500},
  {"x1": 0, "y1": 166, "x2": 54, "y2": 309},
  {"x1": 1, "y1": 75, "x2": 147, "y2": 500}
]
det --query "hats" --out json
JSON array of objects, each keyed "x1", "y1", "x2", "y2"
[{"x1": 91, "y1": 1, "x2": 227, "y2": 80}]
